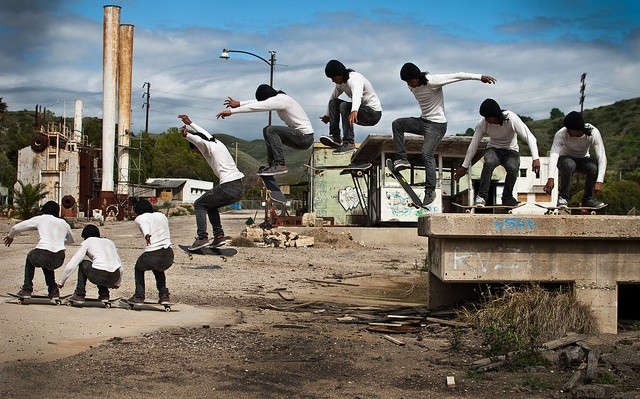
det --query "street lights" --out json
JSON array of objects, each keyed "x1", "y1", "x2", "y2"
[{"x1": 219, "y1": 48, "x2": 277, "y2": 227}]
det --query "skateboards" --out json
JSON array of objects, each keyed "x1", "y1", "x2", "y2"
[
  {"x1": 121, "y1": 299, "x2": 175, "y2": 312},
  {"x1": 259, "y1": 166, "x2": 287, "y2": 205},
  {"x1": 386, "y1": 159, "x2": 430, "y2": 211},
  {"x1": 452, "y1": 202, "x2": 526, "y2": 214},
  {"x1": 7, "y1": 292, "x2": 73, "y2": 306},
  {"x1": 303, "y1": 163, "x2": 372, "y2": 176},
  {"x1": 66, "y1": 298, "x2": 121, "y2": 308},
  {"x1": 178, "y1": 245, "x2": 237, "y2": 262},
  {"x1": 534, "y1": 204, "x2": 608, "y2": 214}
]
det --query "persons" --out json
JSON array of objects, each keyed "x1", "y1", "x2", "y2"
[
  {"x1": 389, "y1": 61, "x2": 498, "y2": 207},
  {"x1": 318, "y1": 57, "x2": 384, "y2": 156},
  {"x1": 544, "y1": 109, "x2": 608, "y2": 208},
  {"x1": 450, "y1": 96, "x2": 542, "y2": 206},
  {"x1": 127, "y1": 198, "x2": 176, "y2": 303},
  {"x1": 57, "y1": 222, "x2": 124, "y2": 302},
  {"x1": 1, "y1": 198, "x2": 77, "y2": 301},
  {"x1": 217, "y1": 84, "x2": 314, "y2": 177},
  {"x1": 179, "y1": 113, "x2": 246, "y2": 251}
]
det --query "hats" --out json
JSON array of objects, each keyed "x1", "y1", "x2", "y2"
[
  {"x1": 256, "y1": 84, "x2": 277, "y2": 101},
  {"x1": 189, "y1": 132, "x2": 208, "y2": 149},
  {"x1": 81, "y1": 225, "x2": 100, "y2": 238},
  {"x1": 325, "y1": 60, "x2": 347, "y2": 77},
  {"x1": 400, "y1": 63, "x2": 421, "y2": 80},
  {"x1": 564, "y1": 111, "x2": 584, "y2": 129},
  {"x1": 480, "y1": 98, "x2": 502, "y2": 117},
  {"x1": 41, "y1": 201, "x2": 59, "y2": 217},
  {"x1": 134, "y1": 200, "x2": 154, "y2": 214}
]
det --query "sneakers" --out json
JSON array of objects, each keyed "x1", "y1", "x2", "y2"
[
  {"x1": 502, "y1": 198, "x2": 522, "y2": 206},
  {"x1": 205, "y1": 236, "x2": 226, "y2": 248},
  {"x1": 261, "y1": 165, "x2": 288, "y2": 176},
  {"x1": 158, "y1": 295, "x2": 170, "y2": 304},
  {"x1": 257, "y1": 166, "x2": 271, "y2": 176},
  {"x1": 319, "y1": 135, "x2": 341, "y2": 148},
  {"x1": 68, "y1": 293, "x2": 85, "y2": 304},
  {"x1": 423, "y1": 189, "x2": 436, "y2": 207},
  {"x1": 99, "y1": 297, "x2": 109, "y2": 302},
  {"x1": 332, "y1": 140, "x2": 356, "y2": 155},
  {"x1": 475, "y1": 195, "x2": 486, "y2": 207},
  {"x1": 16, "y1": 289, "x2": 31, "y2": 299},
  {"x1": 394, "y1": 159, "x2": 412, "y2": 172},
  {"x1": 128, "y1": 295, "x2": 145, "y2": 305},
  {"x1": 47, "y1": 293, "x2": 60, "y2": 299},
  {"x1": 557, "y1": 198, "x2": 568, "y2": 209},
  {"x1": 188, "y1": 237, "x2": 209, "y2": 250},
  {"x1": 581, "y1": 199, "x2": 604, "y2": 208}
]
